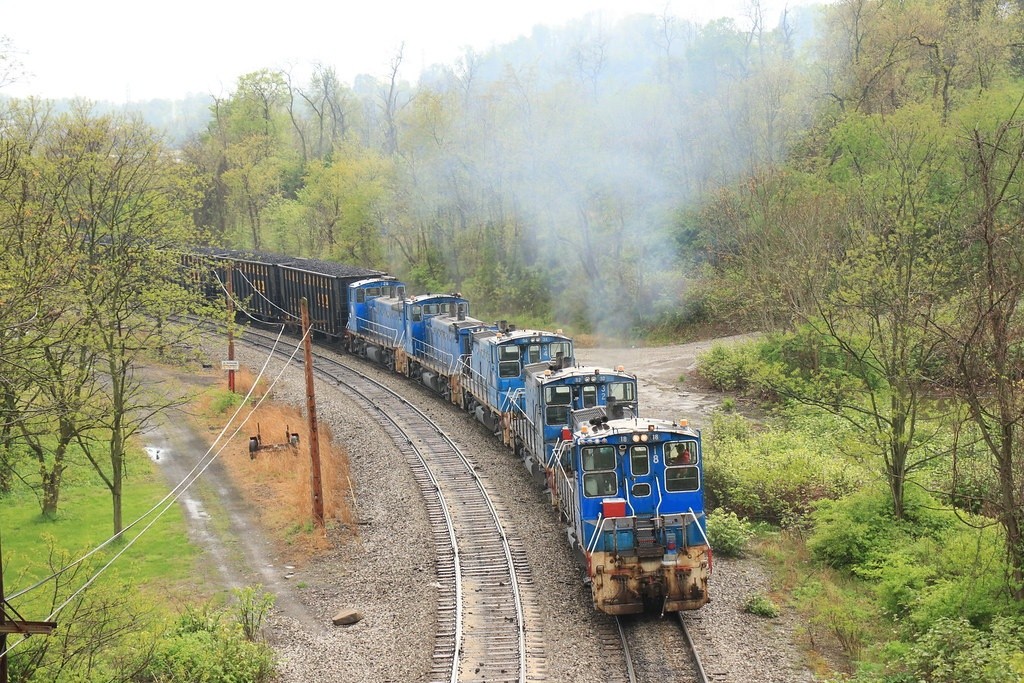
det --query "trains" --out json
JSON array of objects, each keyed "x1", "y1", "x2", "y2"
[{"x1": 93, "y1": 234, "x2": 715, "y2": 619}]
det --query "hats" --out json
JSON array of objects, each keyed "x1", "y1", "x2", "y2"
[{"x1": 675, "y1": 444, "x2": 684, "y2": 448}]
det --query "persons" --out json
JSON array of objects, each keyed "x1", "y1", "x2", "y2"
[{"x1": 672, "y1": 443, "x2": 692, "y2": 491}]
